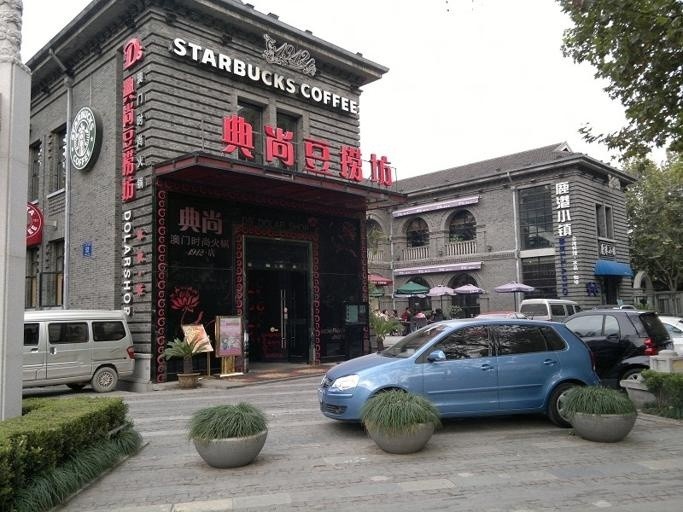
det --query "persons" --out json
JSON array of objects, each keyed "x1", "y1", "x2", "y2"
[{"x1": 374, "y1": 306, "x2": 442, "y2": 336}]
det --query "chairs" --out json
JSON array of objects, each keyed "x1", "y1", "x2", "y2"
[{"x1": 413, "y1": 317, "x2": 426, "y2": 331}]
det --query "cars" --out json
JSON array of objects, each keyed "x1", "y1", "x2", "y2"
[
  {"x1": 592, "y1": 304, "x2": 637, "y2": 310},
  {"x1": 657, "y1": 316, "x2": 683, "y2": 355},
  {"x1": 561, "y1": 309, "x2": 674, "y2": 392},
  {"x1": 474, "y1": 311, "x2": 530, "y2": 320},
  {"x1": 317, "y1": 318, "x2": 602, "y2": 428}
]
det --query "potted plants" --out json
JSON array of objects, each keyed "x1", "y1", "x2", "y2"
[
  {"x1": 559, "y1": 383, "x2": 637, "y2": 442},
  {"x1": 187, "y1": 398, "x2": 268, "y2": 468},
  {"x1": 159, "y1": 333, "x2": 211, "y2": 388},
  {"x1": 359, "y1": 387, "x2": 444, "y2": 453}
]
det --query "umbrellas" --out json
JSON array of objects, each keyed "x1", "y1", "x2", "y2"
[{"x1": 396, "y1": 281, "x2": 535, "y2": 311}]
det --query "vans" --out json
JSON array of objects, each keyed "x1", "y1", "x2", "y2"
[
  {"x1": 519, "y1": 298, "x2": 584, "y2": 322},
  {"x1": 22, "y1": 309, "x2": 136, "y2": 393}
]
